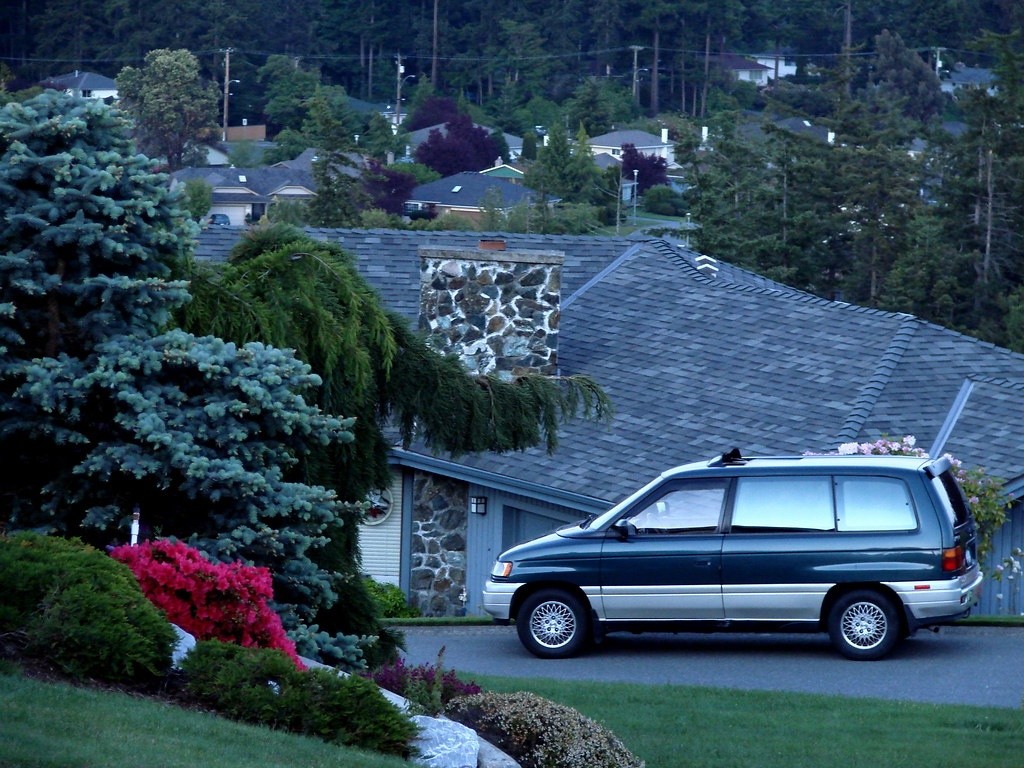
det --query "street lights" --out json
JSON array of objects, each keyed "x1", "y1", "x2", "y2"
[
  {"x1": 222, "y1": 78, "x2": 241, "y2": 141},
  {"x1": 396, "y1": 74, "x2": 415, "y2": 126},
  {"x1": 632, "y1": 67, "x2": 648, "y2": 99}
]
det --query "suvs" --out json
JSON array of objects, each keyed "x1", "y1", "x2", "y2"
[{"x1": 482, "y1": 446, "x2": 985, "y2": 662}]
